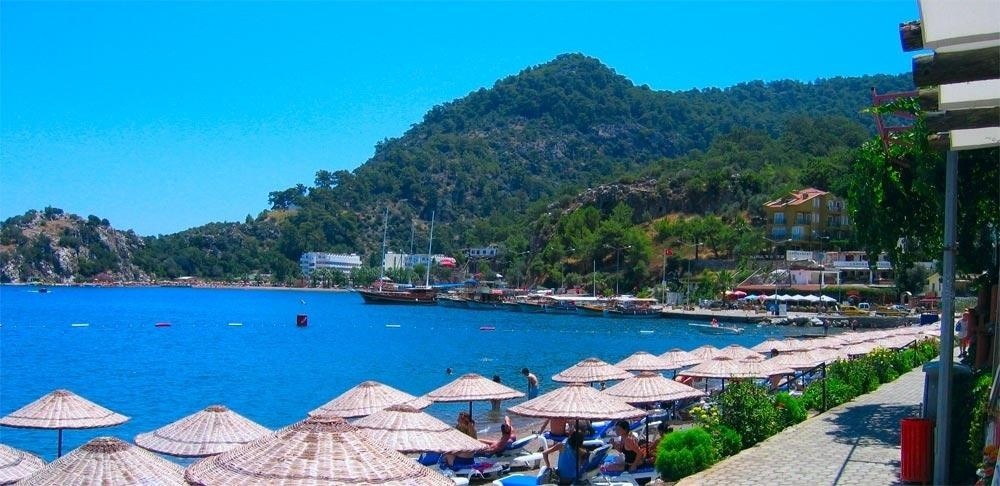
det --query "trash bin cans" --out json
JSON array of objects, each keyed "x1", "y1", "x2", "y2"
[{"x1": 900, "y1": 362, "x2": 973, "y2": 481}]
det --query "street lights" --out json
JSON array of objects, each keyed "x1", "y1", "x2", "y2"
[
  {"x1": 816, "y1": 236, "x2": 829, "y2": 314},
  {"x1": 762, "y1": 236, "x2": 792, "y2": 304},
  {"x1": 677, "y1": 240, "x2": 703, "y2": 305}
]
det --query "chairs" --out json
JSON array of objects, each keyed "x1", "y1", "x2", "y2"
[{"x1": 418, "y1": 371, "x2": 826, "y2": 486}]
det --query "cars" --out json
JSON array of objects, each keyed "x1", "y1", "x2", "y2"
[{"x1": 835, "y1": 302, "x2": 910, "y2": 316}]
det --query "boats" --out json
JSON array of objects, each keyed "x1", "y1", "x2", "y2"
[
  {"x1": 688, "y1": 323, "x2": 744, "y2": 335},
  {"x1": 356, "y1": 207, "x2": 662, "y2": 319}
]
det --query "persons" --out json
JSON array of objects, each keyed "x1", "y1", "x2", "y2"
[
  {"x1": 523, "y1": 368, "x2": 540, "y2": 401},
  {"x1": 442, "y1": 413, "x2": 479, "y2": 466},
  {"x1": 490, "y1": 374, "x2": 502, "y2": 410},
  {"x1": 537, "y1": 416, "x2": 674, "y2": 486},
  {"x1": 956, "y1": 313, "x2": 968, "y2": 358},
  {"x1": 479, "y1": 415, "x2": 519, "y2": 457}
]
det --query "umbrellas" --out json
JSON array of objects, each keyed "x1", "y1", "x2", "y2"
[{"x1": 721, "y1": 290, "x2": 837, "y2": 309}]
